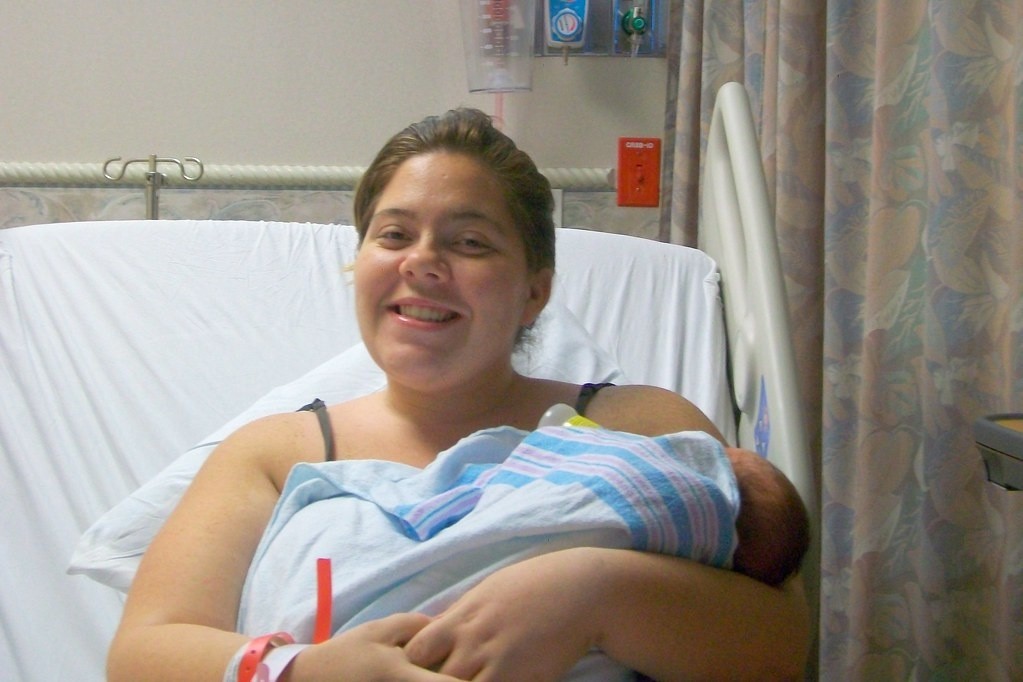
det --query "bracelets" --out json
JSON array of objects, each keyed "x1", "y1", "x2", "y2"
[{"x1": 222, "y1": 559, "x2": 332, "y2": 682}]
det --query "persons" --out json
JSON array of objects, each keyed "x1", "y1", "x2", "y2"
[
  {"x1": 105, "y1": 108, "x2": 810, "y2": 682},
  {"x1": 235, "y1": 426, "x2": 811, "y2": 682}
]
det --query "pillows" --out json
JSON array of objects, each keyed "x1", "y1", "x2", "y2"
[{"x1": 68, "y1": 299, "x2": 605, "y2": 610}]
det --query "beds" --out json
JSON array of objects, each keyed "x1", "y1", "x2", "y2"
[{"x1": 0, "y1": 81, "x2": 817, "y2": 682}]
